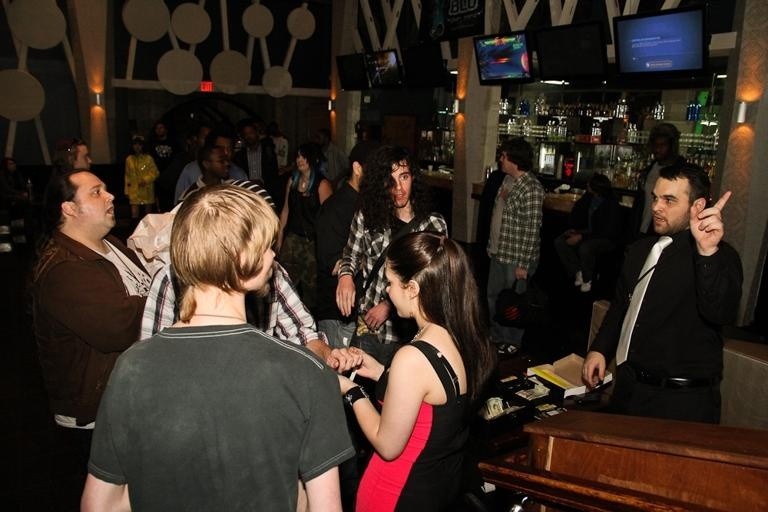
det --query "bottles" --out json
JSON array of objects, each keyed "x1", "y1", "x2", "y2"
[{"x1": 495, "y1": 95, "x2": 717, "y2": 190}]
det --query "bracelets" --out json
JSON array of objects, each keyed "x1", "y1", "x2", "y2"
[{"x1": 343, "y1": 385, "x2": 370, "y2": 405}]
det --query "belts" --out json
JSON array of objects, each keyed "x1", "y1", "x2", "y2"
[{"x1": 645, "y1": 374, "x2": 720, "y2": 389}]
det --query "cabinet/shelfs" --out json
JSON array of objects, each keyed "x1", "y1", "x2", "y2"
[{"x1": 419, "y1": 76, "x2": 723, "y2": 203}]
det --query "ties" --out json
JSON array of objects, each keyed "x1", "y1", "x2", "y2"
[{"x1": 616, "y1": 236, "x2": 673, "y2": 366}]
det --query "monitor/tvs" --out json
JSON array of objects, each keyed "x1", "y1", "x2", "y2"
[
  {"x1": 365, "y1": 49, "x2": 403, "y2": 90},
  {"x1": 335, "y1": 53, "x2": 372, "y2": 91},
  {"x1": 533, "y1": 20, "x2": 609, "y2": 81},
  {"x1": 399, "y1": 42, "x2": 450, "y2": 88},
  {"x1": 613, "y1": 3, "x2": 711, "y2": 89},
  {"x1": 473, "y1": 30, "x2": 534, "y2": 86}
]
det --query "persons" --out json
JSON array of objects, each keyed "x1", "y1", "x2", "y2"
[
  {"x1": 324, "y1": 229, "x2": 497, "y2": 511},
  {"x1": 474, "y1": 135, "x2": 626, "y2": 362},
  {"x1": 30, "y1": 167, "x2": 153, "y2": 511},
  {"x1": 80, "y1": 183, "x2": 359, "y2": 512},
  {"x1": 623, "y1": 122, "x2": 685, "y2": 245},
  {"x1": 52, "y1": 120, "x2": 372, "y2": 374},
  {"x1": 578, "y1": 158, "x2": 744, "y2": 427},
  {"x1": 0, "y1": 155, "x2": 35, "y2": 204}
]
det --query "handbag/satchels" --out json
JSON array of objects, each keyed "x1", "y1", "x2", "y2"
[{"x1": 495, "y1": 275, "x2": 532, "y2": 329}]
